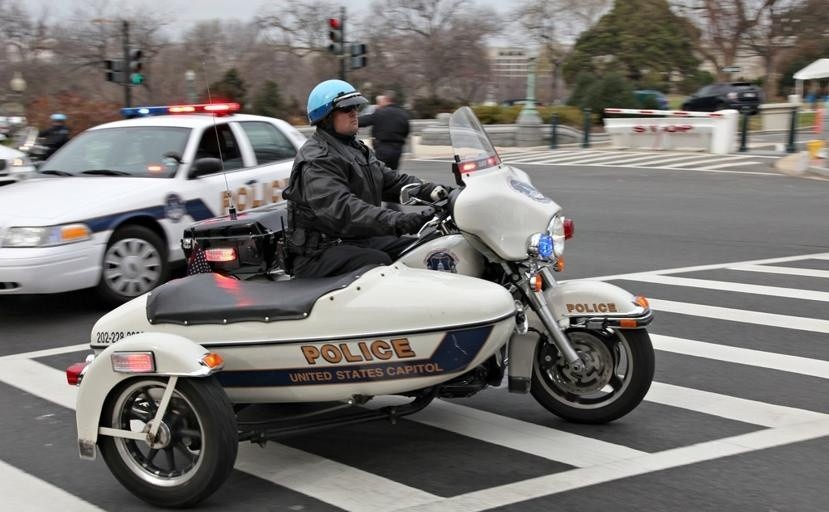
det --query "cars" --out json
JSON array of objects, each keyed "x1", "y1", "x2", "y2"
[{"x1": 634, "y1": 81, "x2": 764, "y2": 115}]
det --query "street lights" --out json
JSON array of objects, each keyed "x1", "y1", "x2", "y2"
[
  {"x1": 516, "y1": 34, "x2": 544, "y2": 124},
  {"x1": 9, "y1": 70, "x2": 27, "y2": 99}
]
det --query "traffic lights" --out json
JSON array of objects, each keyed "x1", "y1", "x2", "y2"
[
  {"x1": 102, "y1": 49, "x2": 146, "y2": 85},
  {"x1": 325, "y1": 17, "x2": 368, "y2": 71}
]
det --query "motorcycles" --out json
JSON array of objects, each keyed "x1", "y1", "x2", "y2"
[{"x1": 66, "y1": 105, "x2": 655, "y2": 508}]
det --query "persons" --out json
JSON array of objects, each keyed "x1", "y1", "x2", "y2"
[
  {"x1": 280, "y1": 78, "x2": 454, "y2": 281},
  {"x1": 359, "y1": 90, "x2": 412, "y2": 171},
  {"x1": 38, "y1": 111, "x2": 71, "y2": 156}
]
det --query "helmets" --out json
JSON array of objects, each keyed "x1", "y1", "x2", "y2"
[
  {"x1": 51, "y1": 114, "x2": 66, "y2": 122},
  {"x1": 306, "y1": 79, "x2": 368, "y2": 126}
]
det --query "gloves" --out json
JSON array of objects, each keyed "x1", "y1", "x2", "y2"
[{"x1": 395, "y1": 212, "x2": 430, "y2": 236}]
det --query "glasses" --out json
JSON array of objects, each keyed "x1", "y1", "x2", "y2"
[{"x1": 334, "y1": 105, "x2": 361, "y2": 114}]
